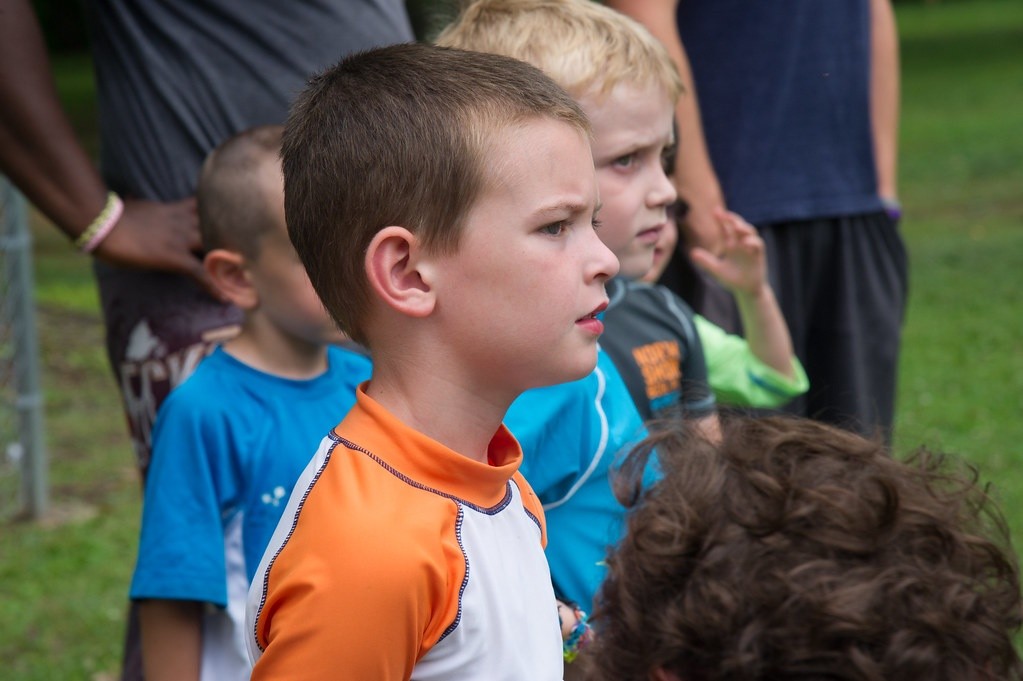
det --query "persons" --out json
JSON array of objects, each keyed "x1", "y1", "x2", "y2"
[
  {"x1": 607, "y1": 0, "x2": 911, "y2": 446},
  {"x1": 429, "y1": 0, "x2": 813, "y2": 664},
  {"x1": 1, "y1": 0, "x2": 417, "y2": 498},
  {"x1": 125, "y1": 125, "x2": 373, "y2": 681},
  {"x1": 237, "y1": 41, "x2": 619, "y2": 681},
  {"x1": 565, "y1": 405, "x2": 1022, "y2": 681}
]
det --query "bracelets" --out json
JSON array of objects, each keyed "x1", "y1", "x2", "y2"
[
  {"x1": 69, "y1": 190, "x2": 126, "y2": 253},
  {"x1": 882, "y1": 202, "x2": 903, "y2": 223},
  {"x1": 564, "y1": 608, "x2": 596, "y2": 663}
]
